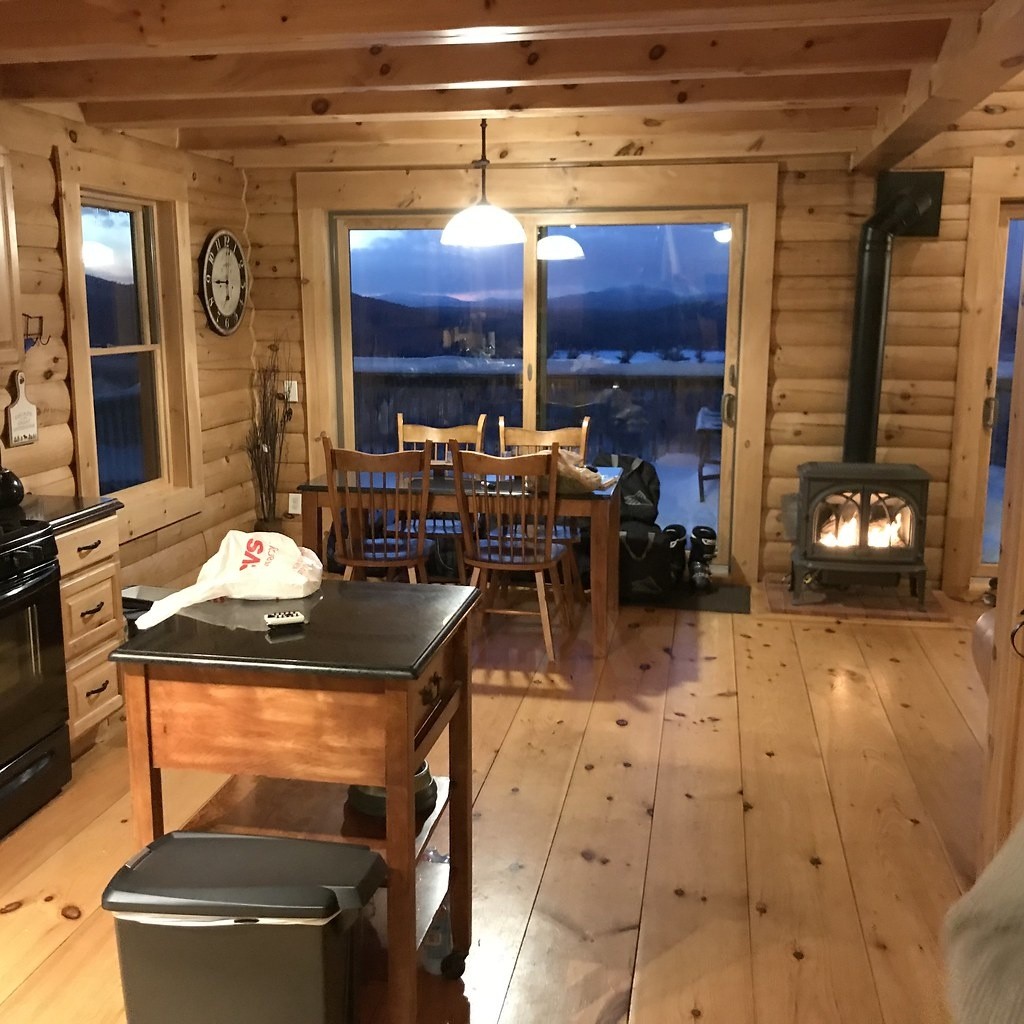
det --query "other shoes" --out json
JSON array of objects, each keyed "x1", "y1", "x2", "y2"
[
  {"x1": 657, "y1": 524, "x2": 688, "y2": 590},
  {"x1": 687, "y1": 526, "x2": 717, "y2": 594}
]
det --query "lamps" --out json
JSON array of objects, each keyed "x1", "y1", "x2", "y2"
[
  {"x1": 439, "y1": 119, "x2": 527, "y2": 249},
  {"x1": 712, "y1": 222, "x2": 733, "y2": 243}
]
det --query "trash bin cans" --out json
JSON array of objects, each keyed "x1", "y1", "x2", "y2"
[{"x1": 99, "y1": 828, "x2": 391, "y2": 1024}]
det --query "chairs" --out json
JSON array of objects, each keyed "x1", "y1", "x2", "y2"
[
  {"x1": 448, "y1": 416, "x2": 591, "y2": 662},
  {"x1": 321, "y1": 413, "x2": 488, "y2": 584},
  {"x1": 695, "y1": 406, "x2": 722, "y2": 503}
]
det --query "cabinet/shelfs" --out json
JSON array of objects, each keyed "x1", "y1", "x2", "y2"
[
  {"x1": 19, "y1": 510, "x2": 127, "y2": 761},
  {"x1": 0, "y1": 519, "x2": 72, "y2": 838},
  {"x1": 109, "y1": 578, "x2": 485, "y2": 1024}
]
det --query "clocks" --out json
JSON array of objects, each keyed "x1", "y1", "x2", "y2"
[{"x1": 199, "y1": 227, "x2": 249, "y2": 336}]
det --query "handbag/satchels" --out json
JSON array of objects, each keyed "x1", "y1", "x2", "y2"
[
  {"x1": 525, "y1": 449, "x2": 601, "y2": 495},
  {"x1": 135, "y1": 530, "x2": 323, "y2": 630},
  {"x1": 326, "y1": 454, "x2": 662, "y2": 589}
]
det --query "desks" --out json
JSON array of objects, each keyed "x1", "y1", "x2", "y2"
[{"x1": 297, "y1": 467, "x2": 623, "y2": 659}]
